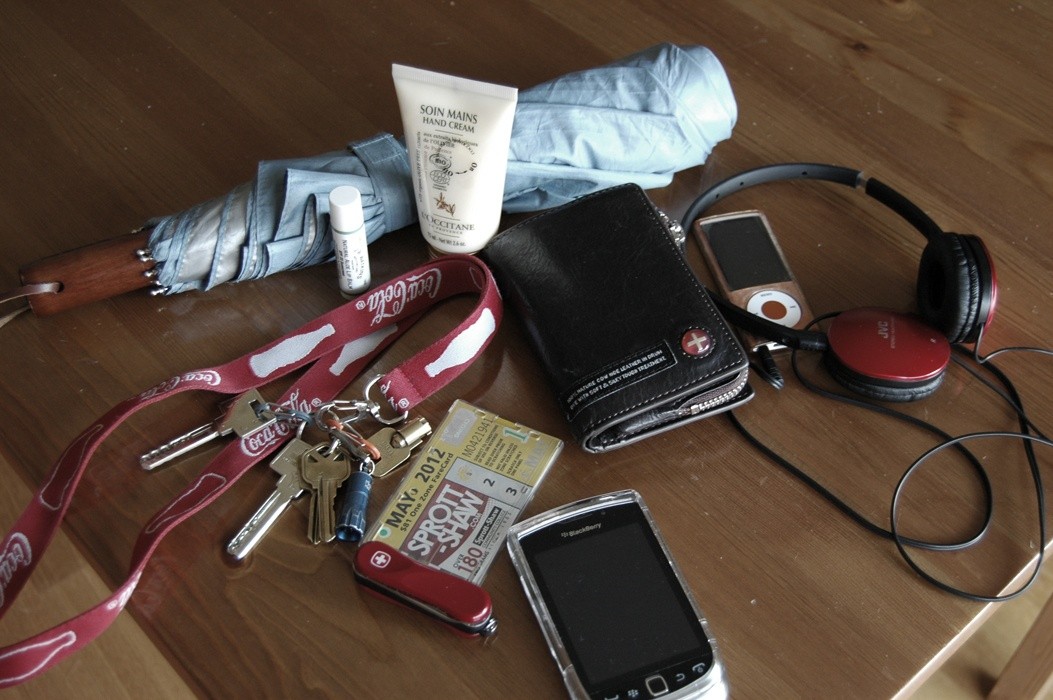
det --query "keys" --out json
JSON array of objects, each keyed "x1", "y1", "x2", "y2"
[
  {"x1": 225, "y1": 438, "x2": 321, "y2": 563},
  {"x1": 296, "y1": 442, "x2": 351, "y2": 545},
  {"x1": 136, "y1": 386, "x2": 275, "y2": 470},
  {"x1": 358, "y1": 414, "x2": 431, "y2": 477},
  {"x1": 301, "y1": 455, "x2": 327, "y2": 545}
]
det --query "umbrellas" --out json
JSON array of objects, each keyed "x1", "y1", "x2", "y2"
[{"x1": 0, "y1": 43, "x2": 738, "y2": 332}]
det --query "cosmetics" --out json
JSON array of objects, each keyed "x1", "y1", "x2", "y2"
[
  {"x1": 329, "y1": 186, "x2": 374, "y2": 300},
  {"x1": 389, "y1": 62, "x2": 519, "y2": 264}
]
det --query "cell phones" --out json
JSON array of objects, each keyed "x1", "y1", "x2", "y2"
[{"x1": 504, "y1": 486, "x2": 726, "y2": 700}]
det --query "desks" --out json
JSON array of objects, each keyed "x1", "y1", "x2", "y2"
[{"x1": 0, "y1": 0, "x2": 1053, "y2": 700}]
